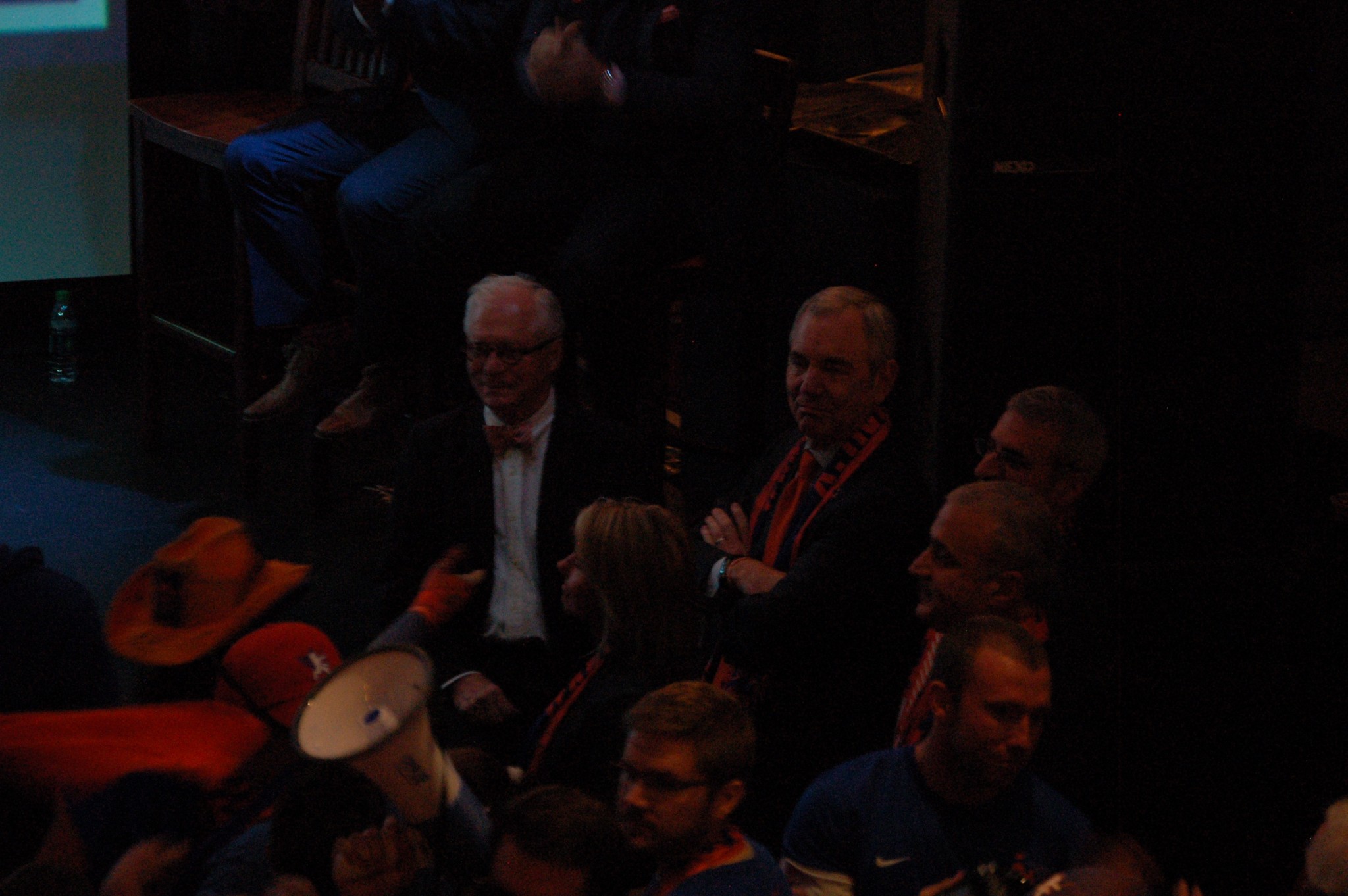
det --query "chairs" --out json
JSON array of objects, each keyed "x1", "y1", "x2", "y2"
[{"x1": 125, "y1": 0, "x2": 1135, "y2": 546}]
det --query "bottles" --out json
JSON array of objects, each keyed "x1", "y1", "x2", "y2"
[{"x1": 48, "y1": 290, "x2": 80, "y2": 383}]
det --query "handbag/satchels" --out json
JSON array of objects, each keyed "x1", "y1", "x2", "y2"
[{"x1": 753, "y1": 49, "x2": 953, "y2": 170}]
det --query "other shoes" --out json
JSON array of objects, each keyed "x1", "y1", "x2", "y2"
[
  {"x1": 314, "y1": 362, "x2": 390, "y2": 440},
  {"x1": 243, "y1": 338, "x2": 335, "y2": 420}
]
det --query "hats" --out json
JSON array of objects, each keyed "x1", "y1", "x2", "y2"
[{"x1": 104, "y1": 516, "x2": 312, "y2": 667}]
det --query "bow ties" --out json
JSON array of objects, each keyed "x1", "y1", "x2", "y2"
[{"x1": 482, "y1": 419, "x2": 538, "y2": 465}]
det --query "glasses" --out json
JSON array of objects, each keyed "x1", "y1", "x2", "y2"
[
  {"x1": 463, "y1": 333, "x2": 563, "y2": 366},
  {"x1": 608, "y1": 759, "x2": 712, "y2": 793}
]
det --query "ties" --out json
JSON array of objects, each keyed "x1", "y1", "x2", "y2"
[
  {"x1": 893, "y1": 628, "x2": 945, "y2": 748},
  {"x1": 711, "y1": 449, "x2": 816, "y2": 691}
]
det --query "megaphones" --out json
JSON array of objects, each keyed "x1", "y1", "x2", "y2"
[{"x1": 293, "y1": 640, "x2": 496, "y2": 876}]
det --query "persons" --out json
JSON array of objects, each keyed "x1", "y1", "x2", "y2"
[
  {"x1": 0, "y1": 0, "x2": 1204, "y2": 896},
  {"x1": 1286, "y1": 793, "x2": 1348, "y2": 896}
]
